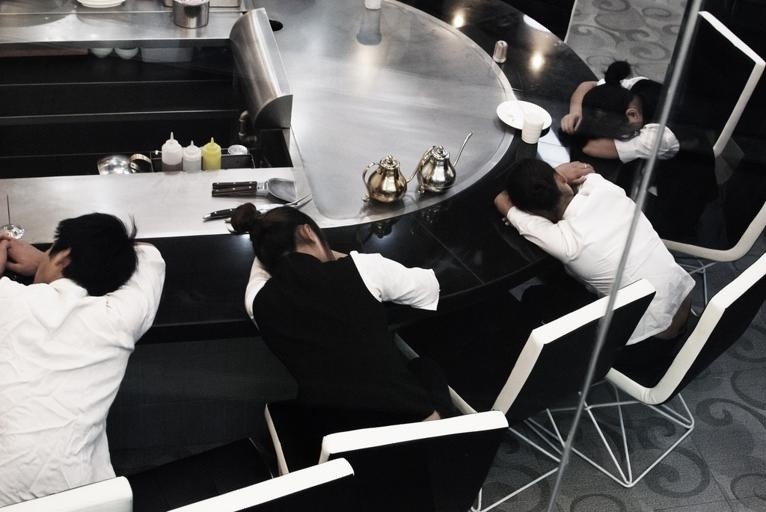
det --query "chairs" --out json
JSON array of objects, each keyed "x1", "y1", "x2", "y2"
[
  {"x1": 395, "y1": 277, "x2": 658, "y2": 512},
  {"x1": 123, "y1": 437, "x2": 356, "y2": 512},
  {"x1": 524, "y1": 251, "x2": 766, "y2": 489},
  {"x1": 0, "y1": 475, "x2": 135, "y2": 512},
  {"x1": 659, "y1": 120, "x2": 766, "y2": 317},
  {"x1": 264, "y1": 398, "x2": 509, "y2": 512},
  {"x1": 677, "y1": 10, "x2": 766, "y2": 158}
]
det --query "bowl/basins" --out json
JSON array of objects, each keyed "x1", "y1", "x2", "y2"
[
  {"x1": 92, "y1": 47, "x2": 139, "y2": 60},
  {"x1": 142, "y1": 47, "x2": 193, "y2": 63}
]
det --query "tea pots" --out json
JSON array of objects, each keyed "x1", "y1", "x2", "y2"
[
  {"x1": 416, "y1": 127, "x2": 473, "y2": 194},
  {"x1": 359, "y1": 153, "x2": 418, "y2": 206}
]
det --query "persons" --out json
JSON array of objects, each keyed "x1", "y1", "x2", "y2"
[
  {"x1": 233, "y1": 201, "x2": 452, "y2": 435},
  {"x1": 0, "y1": 211, "x2": 166, "y2": 503},
  {"x1": 494, "y1": 158, "x2": 697, "y2": 348},
  {"x1": 560, "y1": 62, "x2": 716, "y2": 223}
]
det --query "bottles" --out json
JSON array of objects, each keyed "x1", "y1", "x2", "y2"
[
  {"x1": 493, "y1": 40, "x2": 509, "y2": 66},
  {"x1": 159, "y1": 131, "x2": 223, "y2": 171}
]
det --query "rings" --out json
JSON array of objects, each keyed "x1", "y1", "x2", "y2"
[{"x1": 584, "y1": 164, "x2": 587, "y2": 167}]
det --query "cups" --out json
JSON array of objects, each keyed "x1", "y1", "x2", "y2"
[
  {"x1": 96, "y1": 155, "x2": 131, "y2": 175},
  {"x1": 520, "y1": 112, "x2": 544, "y2": 145},
  {"x1": 172, "y1": 0, "x2": 211, "y2": 29},
  {"x1": 364, "y1": 0, "x2": 382, "y2": 10},
  {"x1": 357, "y1": 9, "x2": 383, "y2": 46}
]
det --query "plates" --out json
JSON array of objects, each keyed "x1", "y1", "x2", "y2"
[
  {"x1": 494, "y1": 100, "x2": 554, "y2": 132},
  {"x1": 76, "y1": 0, "x2": 126, "y2": 8}
]
